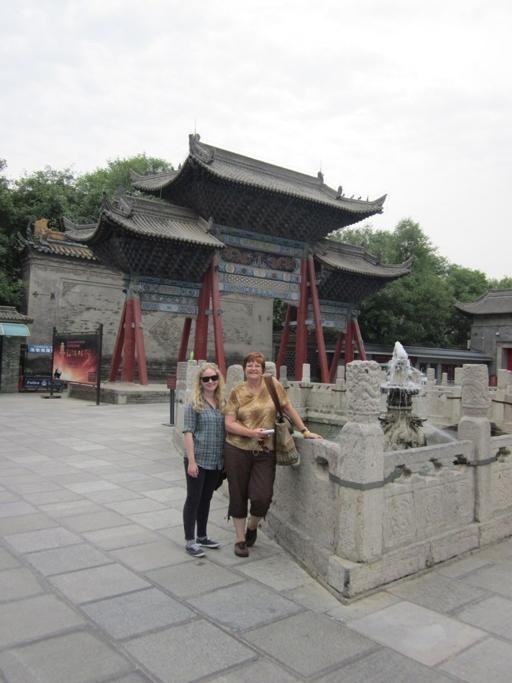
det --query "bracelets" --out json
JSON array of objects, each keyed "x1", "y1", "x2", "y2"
[
  {"x1": 300, "y1": 426, "x2": 308, "y2": 433},
  {"x1": 303, "y1": 430, "x2": 310, "y2": 438}
]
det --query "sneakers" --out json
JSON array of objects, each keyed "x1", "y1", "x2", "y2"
[
  {"x1": 235, "y1": 527, "x2": 257, "y2": 557},
  {"x1": 186, "y1": 544, "x2": 204, "y2": 557},
  {"x1": 196, "y1": 538, "x2": 220, "y2": 547}
]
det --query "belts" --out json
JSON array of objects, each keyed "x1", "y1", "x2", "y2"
[{"x1": 244, "y1": 450, "x2": 264, "y2": 455}]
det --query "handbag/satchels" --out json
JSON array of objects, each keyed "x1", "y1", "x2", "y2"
[{"x1": 276, "y1": 415, "x2": 298, "y2": 465}]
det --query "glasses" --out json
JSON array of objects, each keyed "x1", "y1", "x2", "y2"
[{"x1": 200, "y1": 375, "x2": 218, "y2": 382}]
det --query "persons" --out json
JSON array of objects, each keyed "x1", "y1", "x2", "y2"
[
  {"x1": 223, "y1": 351, "x2": 324, "y2": 558},
  {"x1": 181, "y1": 362, "x2": 248, "y2": 558}
]
